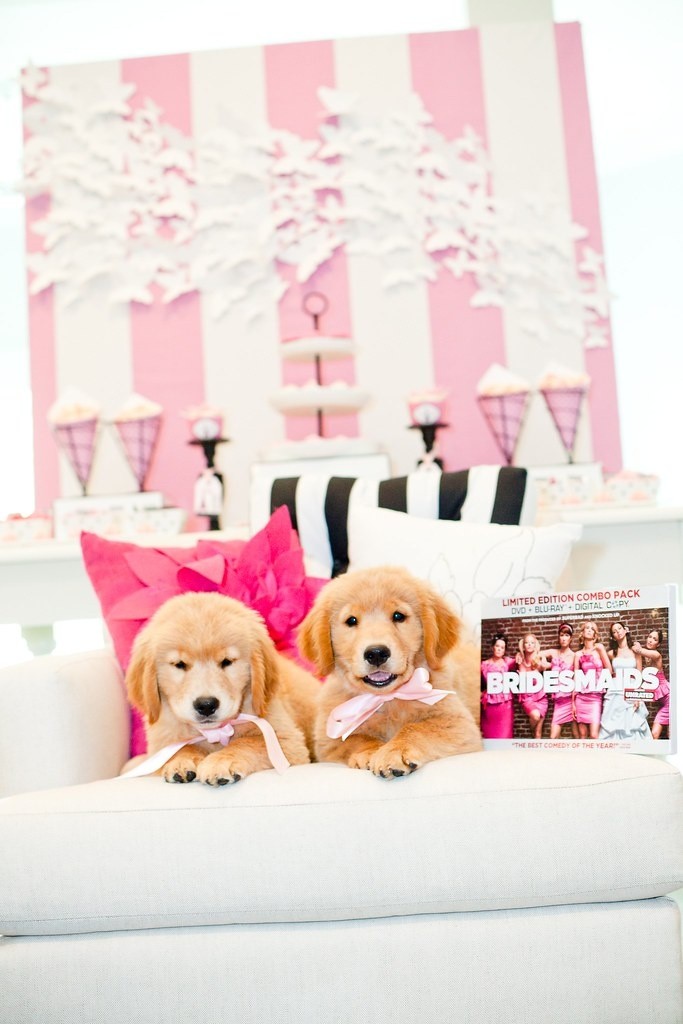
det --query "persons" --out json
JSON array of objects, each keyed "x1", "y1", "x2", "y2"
[
  {"x1": 516, "y1": 634, "x2": 547, "y2": 739},
  {"x1": 632, "y1": 630, "x2": 669, "y2": 739},
  {"x1": 599, "y1": 622, "x2": 655, "y2": 738},
  {"x1": 542, "y1": 623, "x2": 580, "y2": 739},
  {"x1": 481, "y1": 633, "x2": 518, "y2": 739},
  {"x1": 572, "y1": 621, "x2": 613, "y2": 739}
]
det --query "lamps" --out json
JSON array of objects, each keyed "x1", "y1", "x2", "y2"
[
  {"x1": 406, "y1": 401, "x2": 453, "y2": 473},
  {"x1": 184, "y1": 418, "x2": 232, "y2": 533},
  {"x1": 474, "y1": 363, "x2": 534, "y2": 466},
  {"x1": 541, "y1": 366, "x2": 590, "y2": 466},
  {"x1": 106, "y1": 388, "x2": 165, "y2": 497},
  {"x1": 47, "y1": 384, "x2": 102, "y2": 500}
]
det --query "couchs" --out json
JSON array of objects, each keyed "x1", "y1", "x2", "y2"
[{"x1": 0, "y1": 511, "x2": 683, "y2": 1024}]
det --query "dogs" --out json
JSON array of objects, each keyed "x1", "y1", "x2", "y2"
[
  {"x1": 295, "y1": 566, "x2": 485, "y2": 779},
  {"x1": 124, "y1": 593, "x2": 318, "y2": 787}
]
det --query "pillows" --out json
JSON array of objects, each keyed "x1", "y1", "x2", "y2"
[{"x1": 79, "y1": 465, "x2": 584, "y2": 756}]
